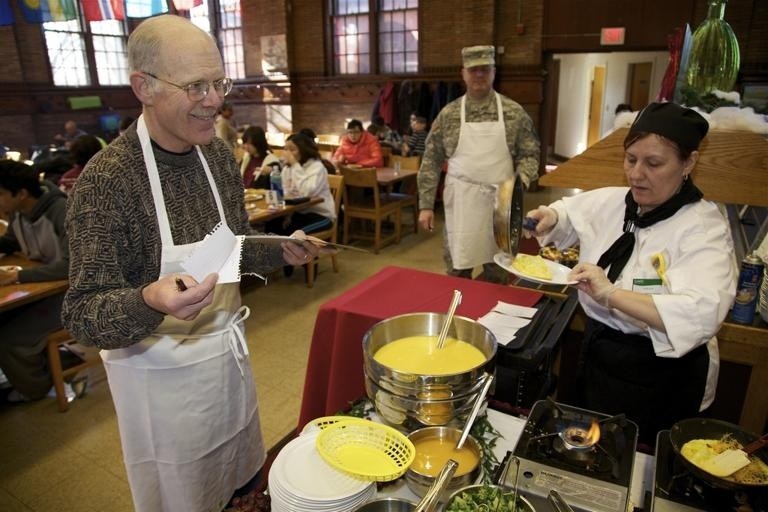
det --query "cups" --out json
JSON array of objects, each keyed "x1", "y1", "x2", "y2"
[{"x1": 395, "y1": 161, "x2": 400, "y2": 176}]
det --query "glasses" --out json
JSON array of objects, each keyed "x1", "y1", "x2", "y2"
[{"x1": 139, "y1": 70, "x2": 234, "y2": 102}]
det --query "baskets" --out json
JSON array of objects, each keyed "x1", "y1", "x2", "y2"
[
  {"x1": 315, "y1": 418, "x2": 417, "y2": 483},
  {"x1": 301, "y1": 415, "x2": 362, "y2": 432}
]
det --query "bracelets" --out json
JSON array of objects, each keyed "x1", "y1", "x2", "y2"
[{"x1": 594, "y1": 283, "x2": 617, "y2": 308}]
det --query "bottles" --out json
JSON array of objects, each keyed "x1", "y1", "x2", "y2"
[{"x1": 270, "y1": 166, "x2": 283, "y2": 206}]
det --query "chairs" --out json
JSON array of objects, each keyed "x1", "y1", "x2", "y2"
[
  {"x1": 340, "y1": 163, "x2": 400, "y2": 257},
  {"x1": 385, "y1": 151, "x2": 420, "y2": 241},
  {"x1": 382, "y1": 145, "x2": 392, "y2": 161},
  {"x1": 46, "y1": 326, "x2": 105, "y2": 415},
  {"x1": 317, "y1": 133, "x2": 339, "y2": 144},
  {"x1": 289, "y1": 174, "x2": 346, "y2": 289}
]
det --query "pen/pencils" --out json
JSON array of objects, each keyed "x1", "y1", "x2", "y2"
[{"x1": 174, "y1": 277, "x2": 186, "y2": 291}]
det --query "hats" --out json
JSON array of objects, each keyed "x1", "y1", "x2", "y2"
[
  {"x1": 629, "y1": 101, "x2": 709, "y2": 151},
  {"x1": 461, "y1": 45, "x2": 495, "y2": 69}
]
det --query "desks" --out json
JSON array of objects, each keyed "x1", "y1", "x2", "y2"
[
  {"x1": 333, "y1": 395, "x2": 655, "y2": 512},
  {"x1": 0, "y1": 253, "x2": 68, "y2": 312},
  {"x1": 243, "y1": 187, "x2": 326, "y2": 228},
  {"x1": 325, "y1": 158, "x2": 418, "y2": 245}
]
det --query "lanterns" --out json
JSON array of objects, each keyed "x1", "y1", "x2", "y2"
[{"x1": 685, "y1": 18, "x2": 741, "y2": 96}]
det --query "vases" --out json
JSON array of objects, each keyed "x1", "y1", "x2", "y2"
[{"x1": 687, "y1": 0, "x2": 742, "y2": 97}]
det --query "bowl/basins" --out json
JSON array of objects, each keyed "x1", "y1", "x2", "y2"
[
  {"x1": 406, "y1": 426, "x2": 484, "y2": 504},
  {"x1": 443, "y1": 485, "x2": 535, "y2": 511}
]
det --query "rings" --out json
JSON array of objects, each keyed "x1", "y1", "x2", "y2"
[{"x1": 304, "y1": 254, "x2": 313, "y2": 264}]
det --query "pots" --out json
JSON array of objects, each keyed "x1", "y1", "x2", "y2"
[
  {"x1": 671, "y1": 419, "x2": 767, "y2": 492},
  {"x1": 494, "y1": 174, "x2": 540, "y2": 255},
  {"x1": 363, "y1": 312, "x2": 499, "y2": 434}
]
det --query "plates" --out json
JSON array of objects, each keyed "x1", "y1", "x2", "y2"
[
  {"x1": 1, "y1": 266, "x2": 23, "y2": 272},
  {"x1": 494, "y1": 253, "x2": 579, "y2": 286},
  {"x1": 269, "y1": 435, "x2": 376, "y2": 511},
  {"x1": 245, "y1": 203, "x2": 256, "y2": 210},
  {"x1": 243, "y1": 194, "x2": 263, "y2": 202}
]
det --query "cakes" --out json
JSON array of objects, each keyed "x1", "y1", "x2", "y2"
[{"x1": 512, "y1": 254, "x2": 553, "y2": 281}]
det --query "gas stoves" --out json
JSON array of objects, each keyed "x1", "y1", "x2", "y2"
[
  {"x1": 492, "y1": 400, "x2": 639, "y2": 511},
  {"x1": 650, "y1": 429, "x2": 749, "y2": 511}
]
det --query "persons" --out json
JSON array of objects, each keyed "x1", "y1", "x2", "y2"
[
  {"x1": 60, "y1": 14, "x2": 341, "y2": 511},
  {"x1": 0, "y1": 159, "x2": 88, "y2": 401},
  {"x1": 518, "y1": 101, "x2": 741, "y2": 445},
  {"x1": 417, "y1": 45, "x2": 542, "y2": 287},
  {"x1": 27, "y1": 116, "x2": 139, "y2": 199},
  {"x1": 213, "y1": 103, "x2": 428, "y2": 192},
  {"x1": 281, "y1": 133, "x2": 337, "y2": 283}
]
